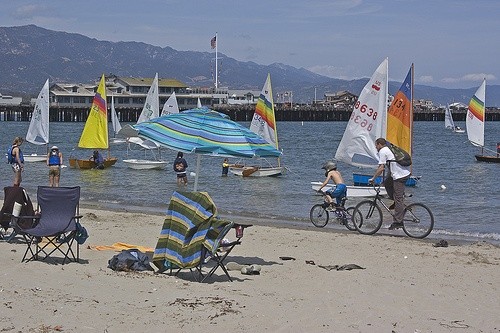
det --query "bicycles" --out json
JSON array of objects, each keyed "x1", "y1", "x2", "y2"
[
  {"x1": 310, "y1": 186, "x2": 362, "y2": 232},
  {"x1": 354, "y1": 178, "x2": 434, "y2": 238}
]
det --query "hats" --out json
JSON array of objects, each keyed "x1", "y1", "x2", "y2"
[{"x1": 50, "y1": 146, "x2": 59, "y2": 150}]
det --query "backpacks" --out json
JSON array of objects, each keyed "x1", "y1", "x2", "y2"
[{"x1": 378, "y1": 142, "x2": 412, "y2": 165}]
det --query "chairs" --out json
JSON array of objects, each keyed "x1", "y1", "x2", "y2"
[
  {"x1": 0, "y1": 187, "x2": 35, "y2": 242},
  {"x1": 19, "y1": 185, "x2": 82, "y2": 265},
  {"x1": 173, "y1": 191, "x2": 253, "y2": 283}
]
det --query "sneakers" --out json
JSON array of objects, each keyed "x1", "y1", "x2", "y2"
[
  {"x1": 326, "y1": 204, "x2": 337, "y2": 211},
  {"x1": 240, "y1": 264, "x2": 262, "y2": 274},
  {"x1": 388, "y1": 221, "x2": 404, "y2": 231},
  {"x1": 226, "y1": 262, "x2": 249, "y2": 271},
  {"x1": 389, "y1": 202, "x2": 396, "y2": 211}
]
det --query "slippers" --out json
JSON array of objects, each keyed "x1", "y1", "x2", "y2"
[
  {"x1": 306, "y1": 260, "x2": 316, "y2": 266},
  {"x1": 279, "y1": 256, "x2": 296, "y2": 260}
]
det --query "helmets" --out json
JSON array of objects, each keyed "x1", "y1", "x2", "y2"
[{"x1": 321, "y1": 161, "x2": 337, "y2": 177}]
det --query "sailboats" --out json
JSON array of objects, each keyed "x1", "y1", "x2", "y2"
[
  {"x1": 160, "y1": 91, "x2": 180, "y2": 117},
  {"x1": 5, "y1": 76, "x2": 54, "y2": 162},
  {"x1": 228, "y1": 73, "x2": 283, "y2": 179},
  {"x1": 67, "y1": 72, "x2": 119, "y2": 170},
  {"x1": 311, "y1": 56, "x2": 390, "y2": 196},
  {"x1": 466, "y1": 78, "x2": 500, "y2": 163},
  {"x1": 108, "y1": 94, "x2": 128, "y2": 144},
  {"x1": 352, "y1": 61, "x2": 422, "y2": 186},
  {"x1": 123, "y1": 70, "x2": 168, "y2": 171},
  {"x1": 444, "y1": 101, "x2": 465, "y2": 133}
]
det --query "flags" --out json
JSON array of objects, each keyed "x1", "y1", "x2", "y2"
[{"x1": 211, "y1": 36, "x2": 216, "y2": 49}]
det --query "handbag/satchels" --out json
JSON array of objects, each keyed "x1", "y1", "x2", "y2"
[{"x1": 108, "y1": 249, "x2": 150, "y2": 272}]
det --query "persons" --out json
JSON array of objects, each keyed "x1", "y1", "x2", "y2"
[
  {"x1": 47, "y1": 145, "x2": 62, "y2": 187},
  {"x1": 174, "y1": 151, "x2": 188, "y2": 186},
  {"x1": 317, "y1": 161, "x2": 347, "y2": 220},
  {"x1": 93, "y1": 150, "x2": 104, "y2": 169},
  {"x1": 7, "y1": 137, "x2": 25, "y2": 187},
  {"x1": 367, "y1": 138, "x2": 411, "y2": 230},
  {"x1": 222, "y1": 158, "x2": 232, "y2": 176}
]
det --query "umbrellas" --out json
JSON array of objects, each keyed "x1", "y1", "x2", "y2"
[{"x1": 133, "y1": 107, "x2": 283, "y2": 192}]
